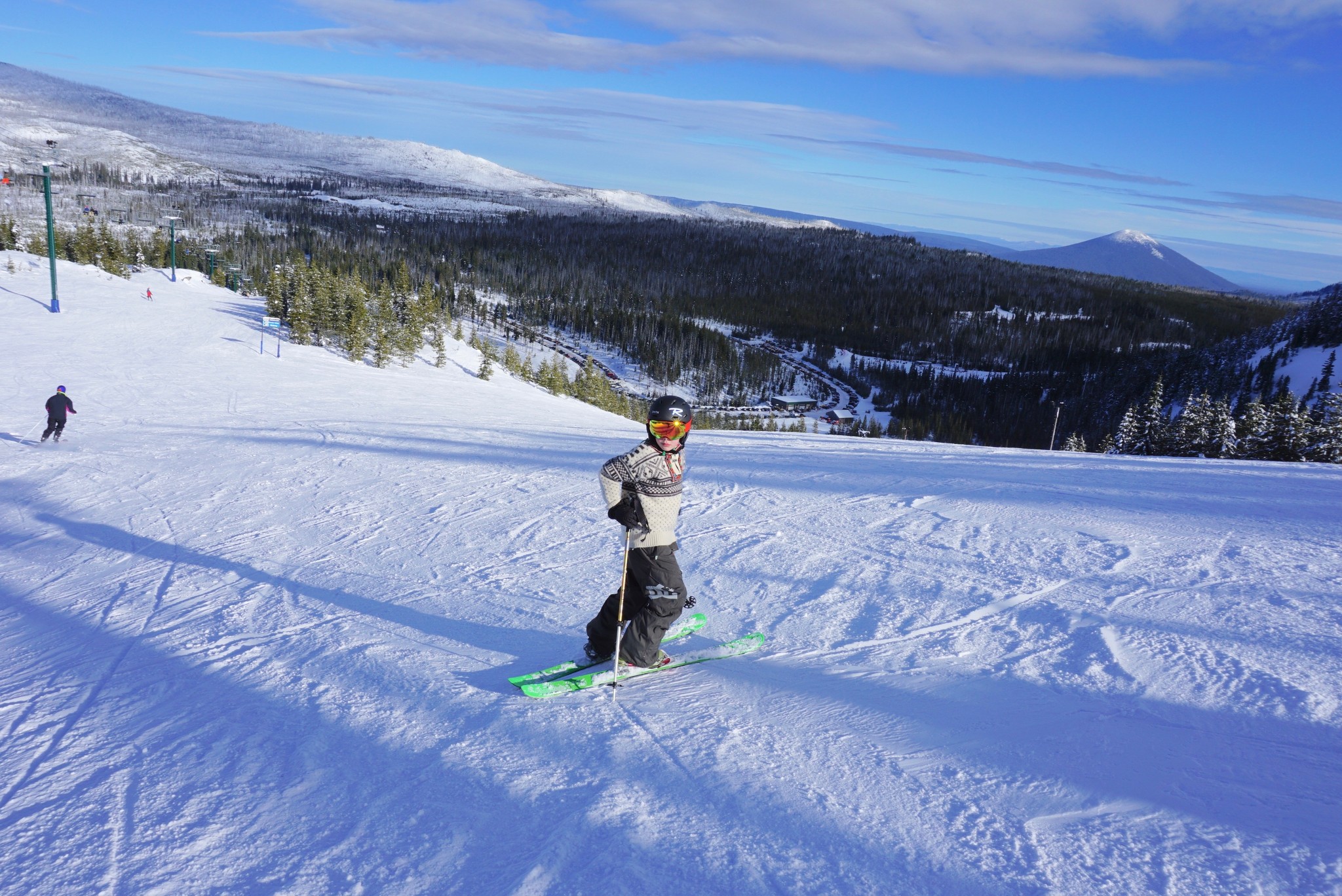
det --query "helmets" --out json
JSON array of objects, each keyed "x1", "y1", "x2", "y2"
[
  {"x1": 57, "y1": 386, "x2": 65, "y2": 393},
  {"x1": 648, "y1": 396, "x2": 692, "y2": 445}
]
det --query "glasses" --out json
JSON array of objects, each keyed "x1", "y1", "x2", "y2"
[{"x1": 650, "y1": 419, "x2": 686, "y2": 441}]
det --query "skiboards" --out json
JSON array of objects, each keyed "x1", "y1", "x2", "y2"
[
  {"x1": 28, "y1": 439, "x2": 68, "y2": 448},
  {"x1": 507, "y1": 612, "x2": 765, "y2": 698}
]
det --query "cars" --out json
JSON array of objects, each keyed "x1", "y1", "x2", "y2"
[
  {"x1": 486, "y1": 311, "x2": 655, "y2": 403},
  {"x1": 692, "y1": 340, "x2": 860, "y2": 419}
]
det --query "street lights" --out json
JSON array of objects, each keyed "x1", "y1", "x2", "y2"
[
  {"x1": 205, "y1": 249, "x2": 219, "y2": 283},
  {"x1": 1049, "y1": 401, "x2": 1064, "y2": 450},
  {"x1": 230, "y1": 268, "x2": 241, "y2": 293},
  {"x1": 26, "y1": 160, "x2": 63, "y2": 312},
  {"x1": 902, "y1": 427, "x2": 910, "y2": 440},
  {"x1": 163, "y1": 216, "x2": 182, "y2": 282}
]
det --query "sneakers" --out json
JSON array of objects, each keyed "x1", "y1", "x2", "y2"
[
  {"x1": 613, "y1": 650, "x2": 670, "y2": 669},
  {"x1": 585, "y1": 639, "x2": 608, "y2": 659}
]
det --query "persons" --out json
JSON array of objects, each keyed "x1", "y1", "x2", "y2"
[
  {"x1": 583, "y1": 395, "x2": 692, "y2": 669},
  {"x1": 41, "y1": 385, "x2": 77, "y2": 442},
  {"x1": 147, "y1": 287, "x2": 153, "y2": 301}
]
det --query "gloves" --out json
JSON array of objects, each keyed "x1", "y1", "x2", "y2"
[{"x1": 608, "y1": 499, "x2": 640, "y2": 529}]
